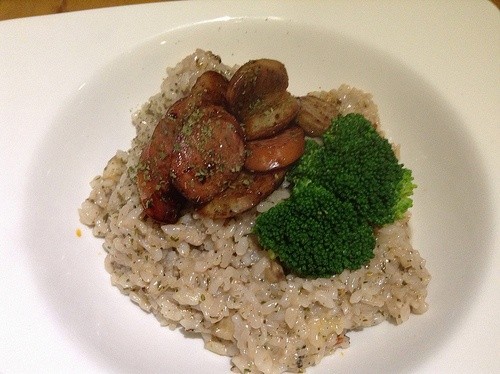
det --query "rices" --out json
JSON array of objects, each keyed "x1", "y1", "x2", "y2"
[{"x1": 76, "y1": 49, "x2": 434, "y2": 374}]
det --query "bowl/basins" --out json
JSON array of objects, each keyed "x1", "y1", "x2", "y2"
[{"x1": 0, "y1": 1, "x2": 498, "y2": 373}]
